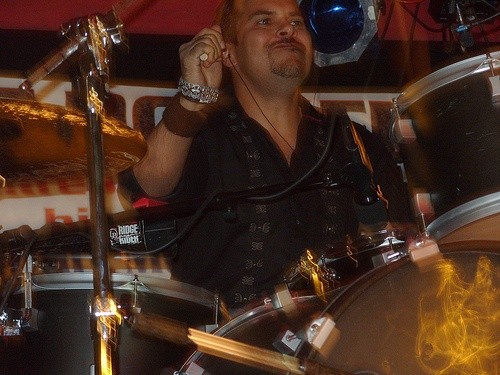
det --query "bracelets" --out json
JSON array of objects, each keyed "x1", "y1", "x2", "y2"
[{"x1": 177, "y1": 75, "x2": 220, "y2": 104}]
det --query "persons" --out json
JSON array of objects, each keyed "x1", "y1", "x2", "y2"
[{"x1": 117, "y1": 0, "x2": 424, "y2": 375}]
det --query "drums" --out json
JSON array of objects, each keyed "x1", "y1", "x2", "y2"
[
  {"x1": 174, "y1": 287, "x2": 327, "y2": 375},
  {"x1": 294, "y1": 250, "x2": 500, "y2": 375},
  {"x1": 0, "y1": 252, "x2": 233, "y2": 375},
  {"x1": 391, "y1": 49, "x2": 500, "y2": 241}
]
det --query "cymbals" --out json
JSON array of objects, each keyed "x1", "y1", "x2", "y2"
[{"x1": 1, "y1": 97, "x2": 148, "y2": 184}]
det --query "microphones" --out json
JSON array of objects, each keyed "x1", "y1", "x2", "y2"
[
  {"x1": 337, "y1": 107, "x2": 388, "y2": 226},
  {"x1": 455, "y1": 4, "x2": 474, "y2": 48}
]
